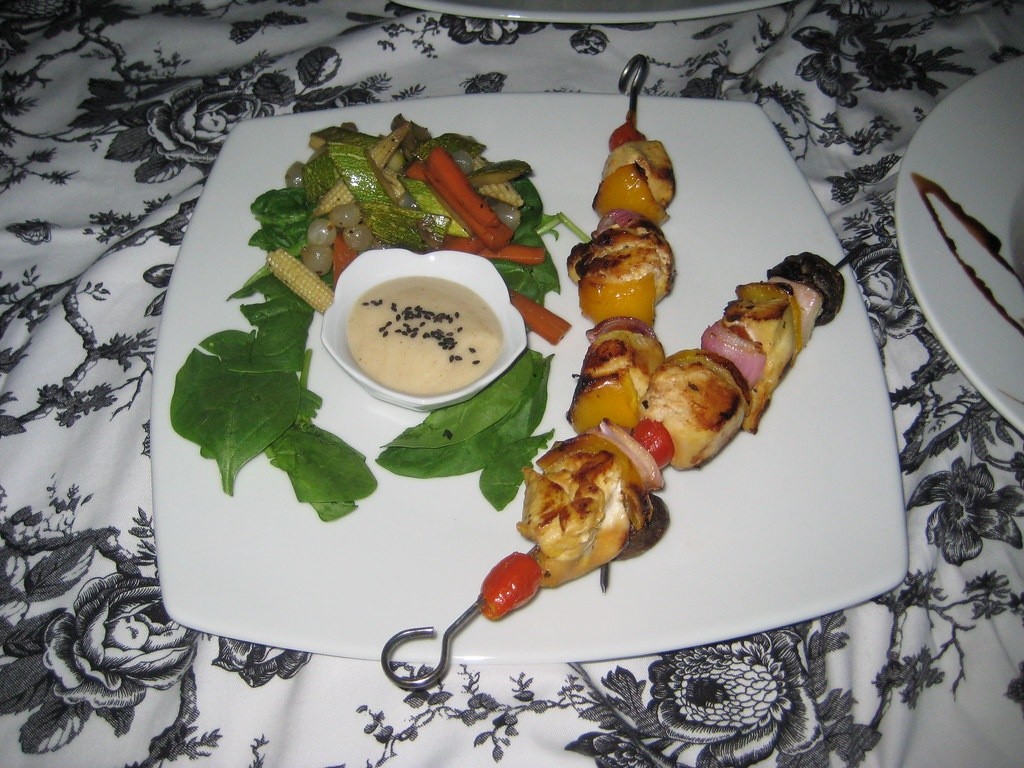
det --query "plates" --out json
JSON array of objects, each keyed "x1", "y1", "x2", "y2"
[
  {"x1": 397, "y1": 2, "x2": 798, "y2": 24},
  {"x1": 149, "y1": 91, "x2": 909, "y2": 665},
  {"x1": 895, "y1": 51, "x2": 1024, "y2": 437}
]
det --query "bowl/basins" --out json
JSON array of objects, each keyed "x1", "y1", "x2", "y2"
[{"x1": 322, "y1": 245, "x2": 531, "y2": 407}]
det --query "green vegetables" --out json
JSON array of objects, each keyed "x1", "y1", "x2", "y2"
[{"x1": 168, "y1": 186, "x2": 572, "y2": 523}]
